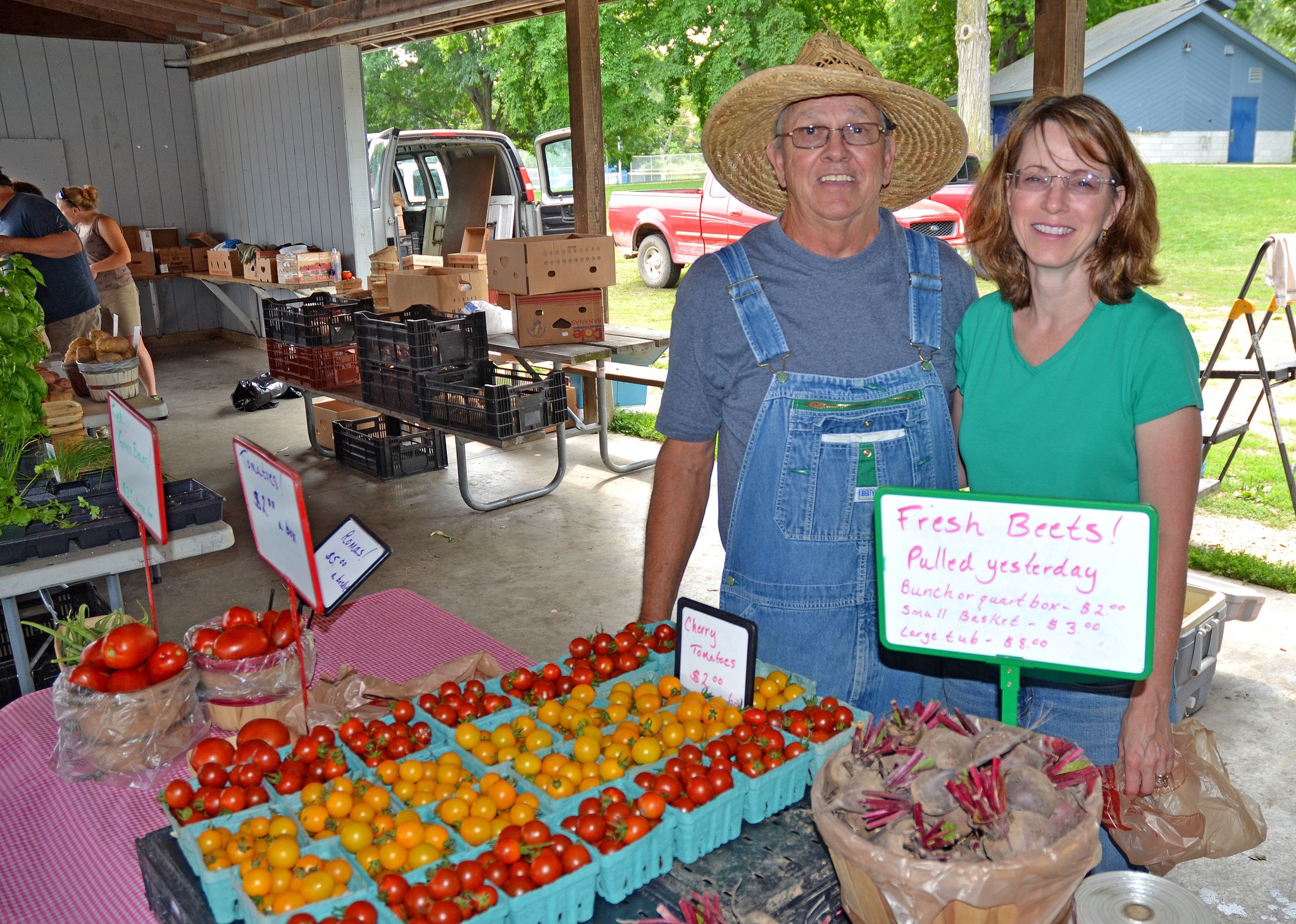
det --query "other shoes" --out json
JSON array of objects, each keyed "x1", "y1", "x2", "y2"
[
  {"x1": 97, "y1": 426, "x2": 110, "y2": 439},
  {"x1": 150, "y1": 394, "x2": 170, "y2": 420}
]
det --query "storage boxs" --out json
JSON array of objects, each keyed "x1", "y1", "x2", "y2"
[
  {"x1": 244, "y1": 259, "x2": 257, "y2": 280},
  {"x1": 276, "y1": 251, "x2": 342, "y2": 285},
  {"x1": 261, "y1": 291, "x2": 374, "y2": 347},
  {"x1": 266, "y1": 338, "x2": 361, "y2": 390},
  {"x1": 312, "y1": 398, "x2": 386, "y2": 450},
  {"x1": 121, "y1": 226, "x2": 222, "y2": 277},
  {"x1": 0, "y1": 582, "x2": 113, "y2": 709},
  {"x1": 256, "y1": 250, "x2": 278, "y2": 282},
  {"x1": 1171, "y1": 573, "x2": 1264, "y2": 721},
  {"x1": 136, "y1": 618, "x2": 872, "y2": 924},
  {"x1": 332, "y1": 413, "x2": 448, "y2": 482},
  {"x1": 207, "y1": 249, "x2": 244, "y2": 276},
  {"x1": 354, "y1": 186, "x2": 616, "y2": 439}
]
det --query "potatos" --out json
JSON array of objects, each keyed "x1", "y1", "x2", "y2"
[
  {"x1": 431, "y1": 388, "x2": 521, "y2": 422},
  {"x1": 63, "y1": 330, "x2": 141, "y2": 364},
  {"x1": 34, "y1": 366, "x2": 73, "y2": 392},
  {"x1": 367, "y1": 342, "x2": 438, "y2": 411}
]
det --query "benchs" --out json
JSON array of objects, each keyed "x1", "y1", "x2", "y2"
[
  {"x1": 274, "y1": 379, "x2": 546, "y2": 511},
  {"x1": 489, "y1": 352, "x2": 667, "y2": 387}
]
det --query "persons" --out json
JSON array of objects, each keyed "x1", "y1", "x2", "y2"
[
  {"x1": 638, "y1": 39, "x2": 982, "y2": 732},
  {"x1": 55, "y1": 184, "x2": 170, "y2": 421},
  {"x1": 950, "y1": 91, "x2": 1204, "y2": 877},
  {"x1": 0, "y1": 166, "x2": 102, "y2": 361}
]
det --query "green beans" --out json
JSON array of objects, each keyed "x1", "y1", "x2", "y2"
[{"x1": 21, "y1": 598, "x2": 149, "y2": 665}]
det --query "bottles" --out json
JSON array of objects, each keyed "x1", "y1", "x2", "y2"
[{"x1": 331, "y1": 248, "x2": 342, "y2": 283}]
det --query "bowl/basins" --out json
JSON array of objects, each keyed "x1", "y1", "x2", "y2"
[{"x1": 342, "y1": 272, "x2": 352, "y2": 279}]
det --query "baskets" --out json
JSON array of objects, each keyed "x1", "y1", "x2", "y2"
[
  {"x1": 1, "y1": 583, "x2": 113, "y2": 710},
  {"x1": 417, "y1": 361, "x2": 569, "y2": 439},
  {"x1": 265, "y1": 338, "x2": 361, "y2": 390},
  {"x1": 331, "y1": 412, "x2": 448, "y2": 481},
  {"x1": 161, "y1": 616, "x2": 872, "y2": 924},
  {"x1": 355, "y1": 302, "x2": 488, "y2": 370},
  {"x1": 261, "y1": 291, "x2": 375, "y2": 348},
  {"x1": 360, "y1": 368, "x2": 471, "y2": 417}
]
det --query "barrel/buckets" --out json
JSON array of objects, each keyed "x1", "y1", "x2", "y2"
[{"x1": 279, "y1": 245, "x2": 308, "y2": 254}]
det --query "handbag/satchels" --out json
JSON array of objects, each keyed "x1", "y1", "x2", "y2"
[{"x1": 1099, "y1": 719, "x2": 1269, "y2": 879}]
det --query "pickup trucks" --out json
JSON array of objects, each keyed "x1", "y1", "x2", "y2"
[
  {"x1": 922, "y1": 151, "x2": 984, "y2": 228},
  {"x1": 608, "y1": 154, "x2": 971, "y2": 289}
]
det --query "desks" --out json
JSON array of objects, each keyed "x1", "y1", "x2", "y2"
[
  {"x1": 132, "y1": 271, "x2": 185, "y2": 336},
  {"x1": 488, "y1": 323, "x2": 671, "y2": 488},
  {"x1": 181, "y1": 271, "x2": 335, "y2": 338},
  {"x1": 0, "y1": 589, "x2": 541, "y2": 924},
  {"x1": 0, "y1": 520, "x2": 234, "y2": 697}
]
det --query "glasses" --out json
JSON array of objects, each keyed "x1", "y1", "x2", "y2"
[
  {"x1": 1005, "y1": 169, "x2": 1116, "y2": 196},
  {"x1": 776, "y1": 121, "x2": 890, "y2": 150},
  {"x1": 58, "y1": 187, "x2": 81, "y2": 211}
]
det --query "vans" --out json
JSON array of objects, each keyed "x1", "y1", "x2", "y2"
[{"x1": 367, "y1": 126, "x2": 575, "y2": 253}]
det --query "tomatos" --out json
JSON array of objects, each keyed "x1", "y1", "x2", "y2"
[
  {"x1": 533, "y1": 670, "x2": 858, "y2": 814},
  {"x1": 501, "y1": 619, "x2": 677, "y2": 708},
  {"x1": 191, "y1": 604, "x2": 305, "y2": 661},
  {"x1": 163, "y1": 719, "x2": 500, "y2": 924},
  {"x1": 339, "y1": 679, "x2": 665, "y2": 900},
  {"x1": 341, "y1": 271, "x2": 352, "y2": 279},
  {"x1": 67, "y1": 622, "x2": 189, "y2": 694}
]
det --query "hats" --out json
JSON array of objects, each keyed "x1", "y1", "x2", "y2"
[{"x1": 701, "y1": 19, "x2": 969, "y2": 217}]
